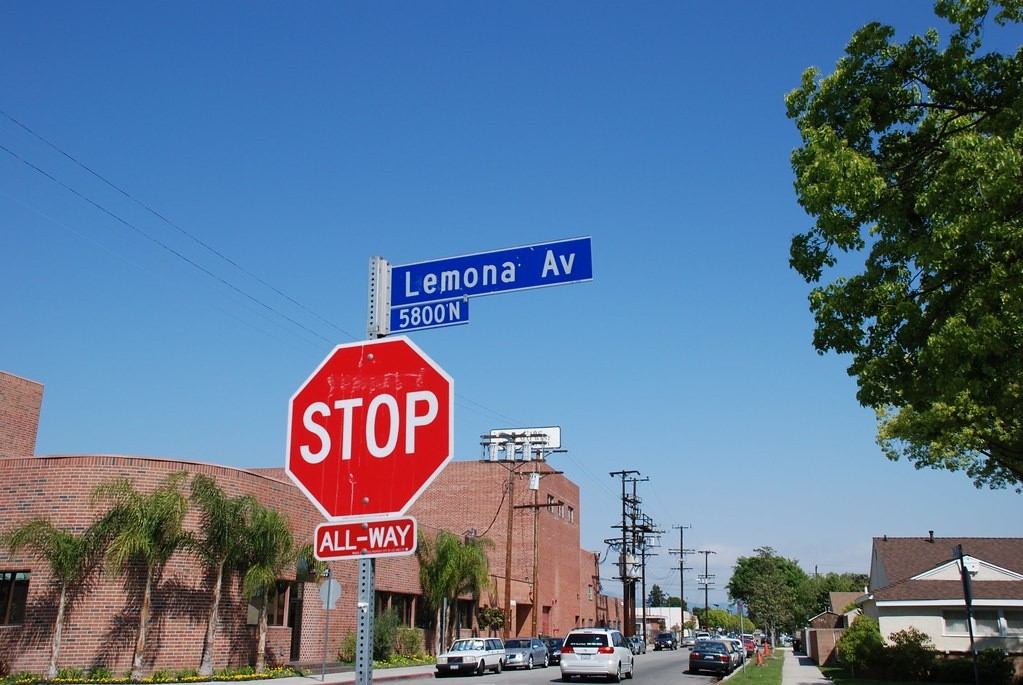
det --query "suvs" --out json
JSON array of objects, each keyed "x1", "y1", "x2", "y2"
[
  {"x1": 653, "y1": 631, "x2": 677, "y2": 651},
  {"x1": 560, "y1": 627, "x2": 635, "y2": 683},
  {"x1": 436, "y1": 638, "x2": 508, "y2": 677}
]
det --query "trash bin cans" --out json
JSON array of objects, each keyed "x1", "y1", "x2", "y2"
[{"x1": 792, "y1": 639, "x2": 800, "y2": 652}]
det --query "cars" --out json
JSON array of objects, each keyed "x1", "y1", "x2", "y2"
[
  {"x1": 541, "y1": 637, "x2": 566, "y2": 666},
  {"x1": 504, "y1": 637, "x2": 549, "y2": 671},
  {"x1": 688, "y1": 640, "x2": 734, "y2": 677},
  {"x1": 681, "y1": 632, "x2": 772, "y2": 670},
  {"x1": 785, "y1": 637, "x2": 794, "y2": 643},
  {"x1": 624, "y1": 637, "x2": 646, "y2": 655}
]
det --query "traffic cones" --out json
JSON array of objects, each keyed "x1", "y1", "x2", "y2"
[
  {"x1": 755, "y1": 650, "x2": 763, "y2": 666},
  {"x1": 763, "y1": 642, "x2": 770, "y2": 656}
]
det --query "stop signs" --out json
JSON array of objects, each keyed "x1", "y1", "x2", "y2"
[{"x1": 284, "y1": 333, "x2": 454, "y2": 524}]
